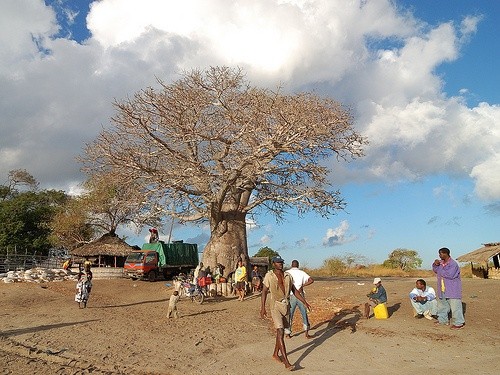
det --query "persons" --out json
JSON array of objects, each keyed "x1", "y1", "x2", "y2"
[
  {"x1": 193, "y1": 262, "x2": 206, "y2": 296},
  {"x1": 431, "y1": 248, "x2": 466, "y2": 332},
  {"x1": 165, "y1": 288, "x2": 183, "y2": 318},
  {"x1": 284, "y1": 260, "x2": 315, "y2": 339},
  {"x1": 212, "y1": 263, "x2": 225, "y2": 284},
  {"x1": 250, "y1": 265, "x2": 263, "y2": 293},
  {"x1": 360, "y1": 278, "x2": 387, "y2": 320},
  {"x1": 233, "y1": 260, "x2": 247, "y2": 302},
  {"x1": 409, "y1": 278, "x2": 438, "y2": 320},
  {"x1": 259, "y1": 256, "x2": 313, "y2": 370},
  {"x1": 63, "y1": 258, "x2": 73, "y2": 270},
  {"x1": 149, "y1": 228, "x2": 159, "y2": 240},
  {"x1": 76, "y1": 261, "x2": 94, "y2": 308}
]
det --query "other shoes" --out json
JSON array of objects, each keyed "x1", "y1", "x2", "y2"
[
  {"x1": 434, "y1": 320, "x2": 449, "y2": 325},
  {"x1": 450, "y1": 325, "x2": 464, "y2": 330}
]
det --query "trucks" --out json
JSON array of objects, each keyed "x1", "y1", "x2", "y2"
[{"x1": 124, "y1": 241, "x2": 199, "y2": 281}]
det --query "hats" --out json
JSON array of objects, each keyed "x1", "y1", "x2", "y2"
[
  {"x1": 271, "y1": 255, "x2": 285, "y2": 263},
  {"x1": 373, "y1": 278, "x2": 382, "y2": 285}
]
